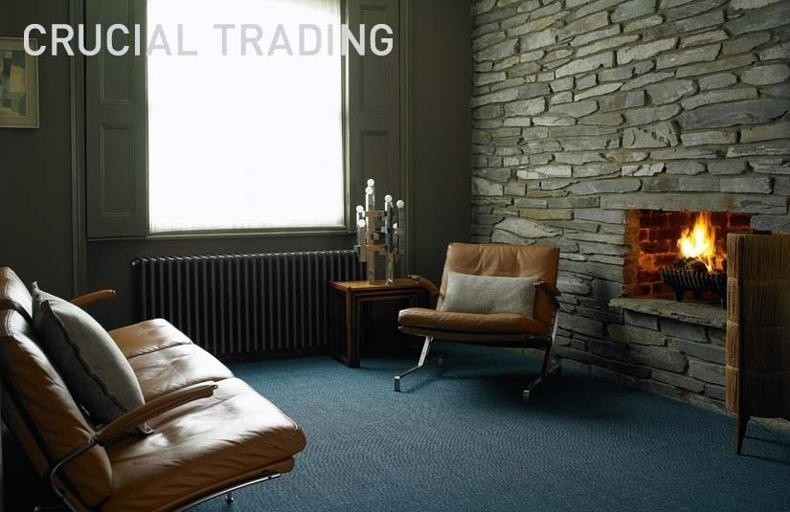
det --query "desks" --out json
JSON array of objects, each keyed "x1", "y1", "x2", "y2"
[{"x1": 328, "y1": 278, "x2": 430, "y2": 368}]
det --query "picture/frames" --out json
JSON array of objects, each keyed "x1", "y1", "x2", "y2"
[{"x1": 0, "y1": 36, "x2": 39, "y2": 129}]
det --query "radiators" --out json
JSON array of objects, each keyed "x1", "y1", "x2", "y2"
[{"x1": 131, "y1": 249, "x2": 361, "y2": 356}]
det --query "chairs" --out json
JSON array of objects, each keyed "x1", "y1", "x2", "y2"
[
  {"x1": 1, "y1": 267, "x2": 306, "y2": 512},
  {"x1": 395, "y1": 242, "x2": 560, "y2": 402}
]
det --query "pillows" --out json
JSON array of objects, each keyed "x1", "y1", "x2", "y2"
[
  {"x1": 32, "y1": 281, "x2": 154, "y2": 435},
  {"x1": 440, "y1": 271, "x2": 540, "y2": 319}
]
